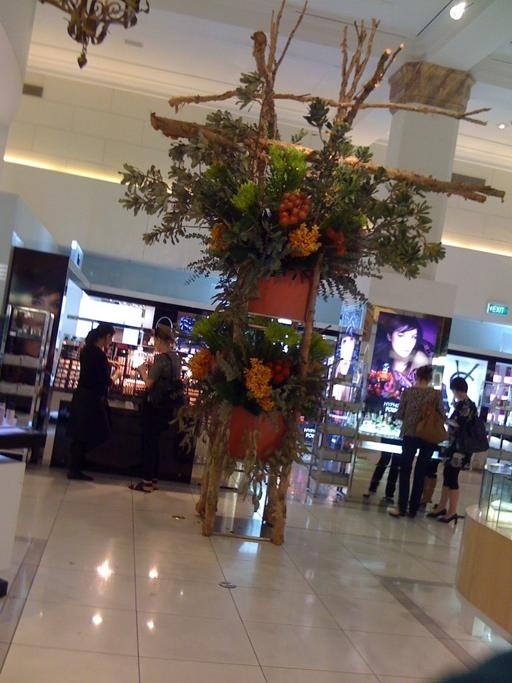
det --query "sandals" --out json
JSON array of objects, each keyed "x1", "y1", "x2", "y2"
[{"x1": 129, "y1": 479, "x2": 159, "y2": 492}]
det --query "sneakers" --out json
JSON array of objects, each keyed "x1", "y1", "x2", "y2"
[
  {"x1": 363, "y1": 492, "x2": 458, "y2": 524},
  {"x1": 66, "y1": 465, "x2": 95, "y2": 481}
]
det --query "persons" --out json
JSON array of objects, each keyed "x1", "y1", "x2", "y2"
[
  {"x1": 371, "y1": 315, "x2": 435, "y2": 373},
  {"x1": 67, "y1": 322, "x2": 124, "y2": 480},
  {"x1": 10, "y1": 274, "x2": 63, "y2": 385},
  {"x1": 128, "y1": 323, "x2": 180, "y2": 493},
  {"x1": 362, "y1": 365, "x2": 478, "y2": 524}
]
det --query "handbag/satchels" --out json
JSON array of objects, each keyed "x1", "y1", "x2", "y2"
[
  {"x1": 455, "y1": 398, "x2": 489, "y2": 453},
  {"x1": 143, "y1": 353, "x2": 173, "y2": 410},
  {"x1": 414, "y1": 390, "x2": 449, "y2": 442}
]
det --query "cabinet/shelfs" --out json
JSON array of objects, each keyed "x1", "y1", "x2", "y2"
[
  {"x1": 478, "y1": 379, "x2": 512, "y2": 472},
  {"x1": 0, "y1": 301, "x2": 116, "y2": 464},
  {"x1": 306, "y1": 360, "x2": 366, "y2": 501}
]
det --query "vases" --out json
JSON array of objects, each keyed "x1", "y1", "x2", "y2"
[{"x1": 206, "y1": 404, "x2": 286, "y2": 464}]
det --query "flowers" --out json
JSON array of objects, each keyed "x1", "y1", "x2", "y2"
[
  {"x1": 190, "y1": 347, "x2": 293, "y2": 415},
  {"x1": 367, "y1": 367, "x2": 409, "y2": 408},
  {"x1": 280, "y1": 190, "x2": 349, "y2": 266},
  {"x1": 210, "y1": 223, "x2": 230, "y2": 255}
]
